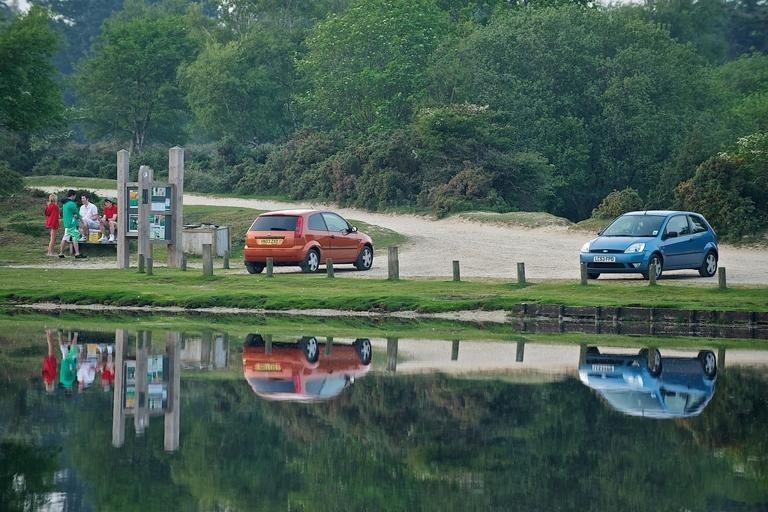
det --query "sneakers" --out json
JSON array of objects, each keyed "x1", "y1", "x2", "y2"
[
  {"x1": 59, "y1": 254, "x2": 67, "y2": 259},
  {"x1": 108, "y1": 234, "x2": 114, "y2": 241},
  {"x1": 79, "y1": 235, "x2": 87, "y2": 242},
  {"x1": 98, "y1": 236, "x2": 108, "y2": 242},
  {"x1": 74, "y1": 253, "x2": 87, "y2": 260}
]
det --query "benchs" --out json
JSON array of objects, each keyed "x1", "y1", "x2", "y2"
[{"x1": 67, "y1": 229, "x2": 117, "y2": 254}]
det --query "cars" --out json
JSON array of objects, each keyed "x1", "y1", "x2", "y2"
[
  {"x1": 242, "y1": 333, "x2": 373, "y2": 405},
  {"x1": 577, "y1": 346, "x2": 718, "y2": 419},
  {"x1": 579, "y1": 210, "x2": 719, "y2": 280},
  {"x1": 244, "y1": 209, "x2": 374, "y2": 274}
]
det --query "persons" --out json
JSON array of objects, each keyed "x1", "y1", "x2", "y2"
[
  {"x1": 78, "y1": 194, "x2": 99, "y2": 242},
  {"x1": 45, "y1": 194, "x2": 61, "y2": 257},
  {"x1": 98, "y1": 198, "x2": 117, "y2": 242},
  {"x1": 58, "y1": 189, "x2": 87, "y2": 258},
  {"x1": 41, "y1": 329, "x2": 115, "y2": 397}
]
